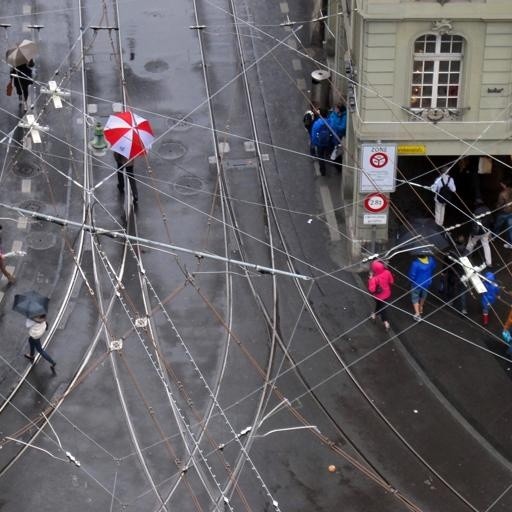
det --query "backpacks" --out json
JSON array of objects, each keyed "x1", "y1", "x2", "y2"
[
  {"x1": 438, "y1": 176, "x2": 452, "y2": 204},
  {"x1": 319, "y1": 125, "x2": 332, "y2": 144}
]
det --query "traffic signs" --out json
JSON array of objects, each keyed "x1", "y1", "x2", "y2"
[
  {"x1": 361, "y1": 143, "x2": 395, "y2": 192},
  {"x1": 363, "y1": 192, "x2": 389, "y2": 212}
]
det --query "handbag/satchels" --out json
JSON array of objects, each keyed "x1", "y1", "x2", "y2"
[{"x1": 6, "y1": 83, "x2": 12, "y2": 95}]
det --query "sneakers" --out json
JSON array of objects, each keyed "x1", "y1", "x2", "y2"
[
  {"x1": 24, "y1": 353, "x2": 34, "y2": 360},
  {"x1": 371, "y1": 309, "x2": 489, "y2": 333}
]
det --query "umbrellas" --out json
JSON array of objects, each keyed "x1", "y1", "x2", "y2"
[
  {"x1": 12, "y1": 290, "x2": 50, "y2": 317},
  {"x1": 400, "y1": 217, "x2": 450, "y2": 255},
  {"x1": 5, "y1": 38, "x2": 37, "y2": 66},
  {"x1": 102, "y1": 111, "x2": 155, "y2": 160}
]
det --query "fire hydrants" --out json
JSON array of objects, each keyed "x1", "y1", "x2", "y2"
[{"x1": 91, "y1": 122, "x2": 107, "y2": 153}]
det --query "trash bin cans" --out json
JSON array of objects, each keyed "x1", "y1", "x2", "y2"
[{"x1": 311, "y1": 69, "x2": 331, "y2": 114}]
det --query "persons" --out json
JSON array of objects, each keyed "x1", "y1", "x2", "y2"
[
  {"x1": 310, "y1": 108, "x2": 332, "y2": 176},
  {"x1": 469, "y1": 156, "x2": 484, "y2": 205},
  {"x1": 428, "y1": 167, "x2": 457, "y2": 227},
  {"x1": 442, "y1": 236, "x2": 472, "y2": 314},
  {"x1": 462, "y1": 200, "x2": 495, "y2": 269},
  {"x1": 114, "y1": 151, "x2": 139, "y2": 203},
  {"x1": 25, "y1": 313, "x2": 56, "y2": 369},
  {"x1": 302, "y1": 102, "x2": 327, "y2": 157},
  {"x1": 329, "y1": 104, "x2": 347, "y2": 159},
  {"x1": 407, "y1": 253, "x2": 437, "y2": 322},
  {"x1": 503, "y1": 308, "x2": 512, "y2": 371},
  {"x1": 479, "y1": 272, "x2": 499, "y2": 325},
  {"x1": 491, "y1": 180, "x2": 512, "y2": 250},
  {"x1": 9, "y1": 65, "x2": 34, "y2": 111},
  {"x1": 0, "y1": 246, "x2": 16, "y2": 288},
  {"x1": 368, "y1": 261, "x2": 394, "y2": 329}
]
